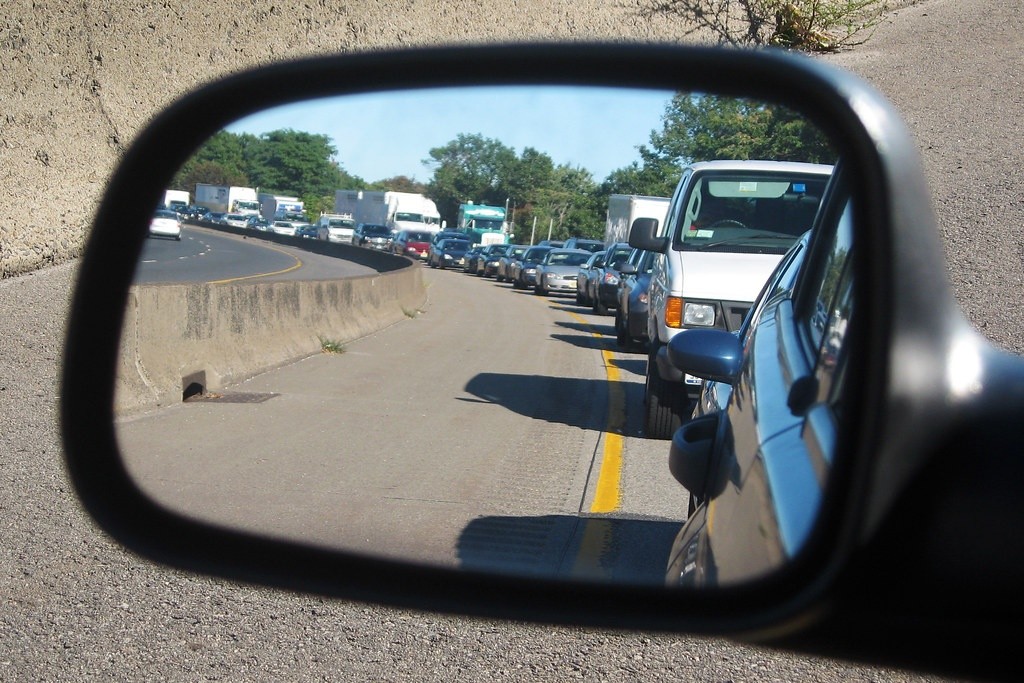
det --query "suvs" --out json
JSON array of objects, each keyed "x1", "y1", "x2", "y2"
[{"x1": 630, "y1": 161, "x2": 835, "y2": 439}]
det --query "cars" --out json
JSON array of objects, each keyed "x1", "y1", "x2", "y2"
[
  {"x1": 157, "y1": 205, "x2": 318, "y2": 238},
  {"x1": 313, "y1": 211, "x2": 472, "y2": 270},
  {"x1": 615, "y1": 249, "x2": 653, "y2": 348},
  {"x1": 148, "y1": 210, "x2": 182, "y2": 240},
  {"x1": 463, "y1": 237, "x2": 634, "y2": 315}
]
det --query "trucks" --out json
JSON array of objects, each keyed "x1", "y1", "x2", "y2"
[
  {"x1": 164, "y1": 181, "x2": 306, "y2": 220},
  {"x1": 334, "y1": 189, "x2": 447, "y2": 235},
  {"x1": 457, "y1": 200, "x2": 514, "y2": 245},
  {"x1": 603, "y1": 194, "x2": 671, "y2": 251}
]
